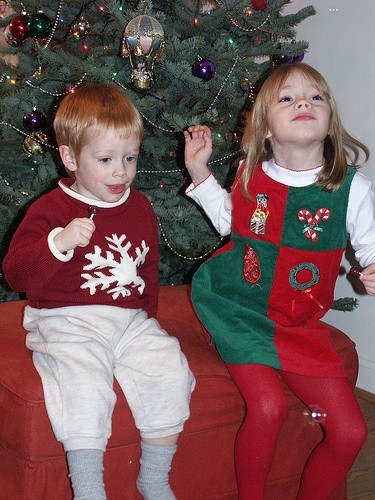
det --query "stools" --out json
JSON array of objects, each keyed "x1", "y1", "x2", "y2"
[{"x1": 1, "y1": 283, "x2": 360, "y2": 499}]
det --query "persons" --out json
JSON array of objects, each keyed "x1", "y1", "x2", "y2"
[
  {"x1": 0, "y1": 81, "x2": 200, "y2": 500},
  {"x1": 180, "y1": 58, "x2": 375, "y2": 500}
]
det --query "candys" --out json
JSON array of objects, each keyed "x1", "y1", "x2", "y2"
[
  {"x1": 88, "y1": 205, "x2": 100, "y2": 216},
  {"x1": 350, "y1": 265, "x2": 360, "y2": 277}
]
